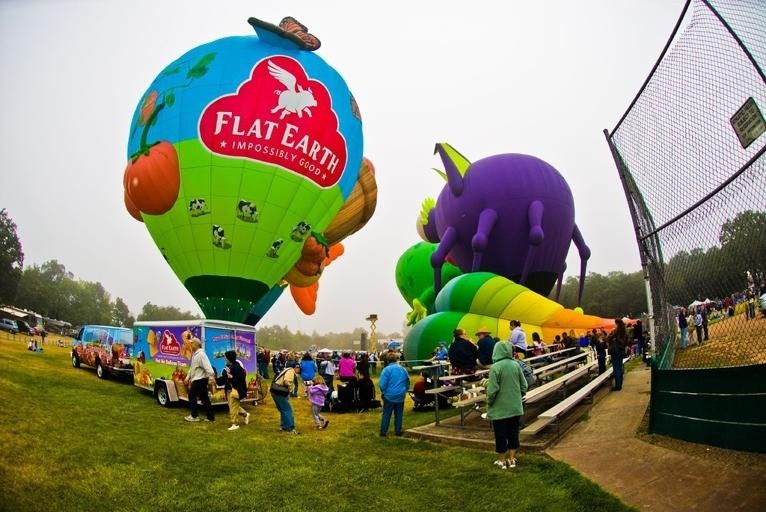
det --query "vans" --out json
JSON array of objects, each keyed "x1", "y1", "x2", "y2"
[
  {"x1": 0, "y1": 318, "x2": 18, "y2": 332},
  {"x1": 70, "y1": 324, "x2": 134, "y2": 378}
]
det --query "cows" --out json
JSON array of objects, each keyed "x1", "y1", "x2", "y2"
[
  {"x1": 211, "y1": 224, "x2": 227, "y2": 248},
  {"x1": 291, "y1": 222, "x2": 311, "y2": 240},
  {"x1": 188, "y1": 199, "x2": 208, "y2": 213},
  {"x1": 237, "y1": 199, "x2": 259, "y2": 223},
  {"x1": 267, "y1": 239, "x2": 284, "y2": 256}
]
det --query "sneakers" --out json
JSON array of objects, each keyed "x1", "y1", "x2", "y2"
[
  {"x1": 204, "y1": 419, "x2": 210, "y2": 422},
  {"x1": 228, "y1": 424, "x2": 240, "y2": 430},
  {"x1": 494, "y1": 459, "x2": 508, "y2": 470},
  {"x1": 312, "y1": 425, "x2": 323, "y2": 431},
  {"x1": 506, "y1": 457, "x2": 517, "y2": 468},
  {"x1": 184, "y1": 414, "x2": 201, "y2": 422},
  {"x1": 243, "y1": 413, "x2": 250, "y2": 424},
  {"x1": 322, "y1": 419, "x2": 329, "y2": 428}
]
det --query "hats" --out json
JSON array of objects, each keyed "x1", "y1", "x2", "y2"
[
  {"x1": 475, "y1": 326, "x2": 492, "y2": 336},
  {"x1": 188, "y1": 338, "x2": 201, "y2": 345},
  {"x1": 225, "y1": 351, "x2": 236, "y2": 362}
]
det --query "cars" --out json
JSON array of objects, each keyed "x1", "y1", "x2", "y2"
[{"x1": 17, "y1": 320, "x2": 35, "y2": 336}]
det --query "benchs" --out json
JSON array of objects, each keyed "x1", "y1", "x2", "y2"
[{"x1": 413, "y1": 344, "x2": 632, "y2": 436}]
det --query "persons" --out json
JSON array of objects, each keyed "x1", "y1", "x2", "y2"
[
  {"x1": 413, "y1": 319, "x2": 615, "y2": 413},
  {"x1": 56, "y1": 340, "x2": 68, "y2": 348},
  {"x1": 28, "y1": 338, "x2": 37, "y2": 352},
  {"x1": 182, "y1": 337, "x2": 216, "y2": 422},
  {"x1": 602, "y1": 318, "x2": 630, "y2": 392},
  {"x1": 40, "y1": 329, "x2": 46, "y2": 343},
  {"x1": 269, "y1": 363, "x2": 302, "y2": 434},
  {"x1": 33, "y1": 340, "x2": 42, "y2": 353},
  {"x1": 305, "y1": 375, "x2": 330, "y2": 431},
  {"x1": 255, "y1": 346, "x2": 406, "y2": 413},
  {"x1": 626, "y1": 318, "x2": 653, "y2": 363},
  {"x1": 209, "y1": 349, "x2": 251, "y2": 430},
  {"x1": 378, "y1": 351, "x2": 410, "y2": 436},
  {"x1": 674, "y1": 280, "x2": 766, "y2": 351},
  {"x1": 484, "y1": 340, "x2": 528, "y2": 469}
]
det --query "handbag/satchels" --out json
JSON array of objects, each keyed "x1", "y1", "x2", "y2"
[
  {"x1": 608, "y1": 340, "x2": 620, "y2": 355},
  {"x1": 229, "y1": 388, "x2": 240, "y2": 400},
  {"x1": 270, "y1": 381, "x2": 289, "y2": 397}
]
